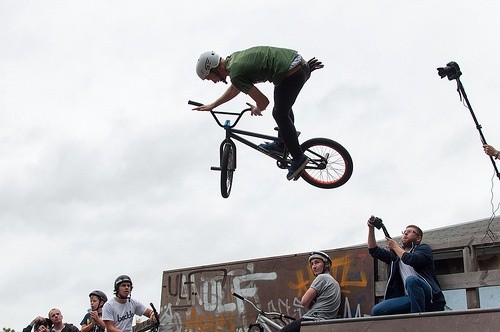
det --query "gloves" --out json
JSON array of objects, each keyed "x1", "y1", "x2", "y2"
[{"x1": 307, "y1": 57, "x2": 324, "y2": 71}]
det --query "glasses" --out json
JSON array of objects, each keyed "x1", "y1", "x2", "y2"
[{"x1": 401, "y1": 229, "x2": 419, "y2": 236}]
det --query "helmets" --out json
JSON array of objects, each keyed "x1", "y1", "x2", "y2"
[
  {"x1": 114, "y1": 275, "x2": 133, "y2": 291},
  {"x1": 308, "y1": 251, "x2": 332, "y2": 271},
  {"x1": 196, "y1": 50, "x2": 222, "y2": 80},
  {"x1": 89, "y1": 290, "x2": 107, "y2": 302}
]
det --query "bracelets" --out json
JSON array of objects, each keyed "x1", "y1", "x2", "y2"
[{"x1": 495, "y1": 151, "x2": 500, "y2": 159}]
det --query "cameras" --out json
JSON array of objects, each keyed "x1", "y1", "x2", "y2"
[
  {"x1": 437, "y1": 61, "x2": 463, "y2": 81},
  {"x1": 370, "y1": 215, "x2": 383, "y2": 230}
]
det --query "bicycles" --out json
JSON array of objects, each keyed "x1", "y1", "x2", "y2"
[
  {"x1": 232, "y1": 292, "x2": 296, "y2": 332},
  {"x1": 187, "y1": 100, "x2": 354, "y2": 199}
]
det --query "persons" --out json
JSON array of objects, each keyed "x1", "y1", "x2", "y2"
[
  {"x1": 191, "y1": 46, "x2": 312, "y2": 180},
  {"x1": 482, "y1": 144, "x2": 500, "y2": 160},
  {"x1": 278, "y1": 251, "x2": 341, "y2": 332},
  {"x1": 367, "y1": 215, "x2": 447, "y2": 316},
  {"x1": 101, "y1": 274, "x2": 156, "y2": 332},
  {"x1": 48, "y1": 308, "x2": 80, "y2": 332},
  {"x1": 23, "y1": 316, "x2": 53, "y2": 332},
  {"x1": 80, "y1": 290, "x2": 108, "y2": 332}
]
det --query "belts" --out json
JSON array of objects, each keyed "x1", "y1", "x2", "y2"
[{"x1": 286, "y1": 58, "x2": 305, "y2": 77}]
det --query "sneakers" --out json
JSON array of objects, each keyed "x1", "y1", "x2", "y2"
[
  {"x1": 259, "y1": 141, "x2": 289, "y2": 156},
  {"x1": 287, "y1": 155, "x2": 310, "y2": 181}
]
